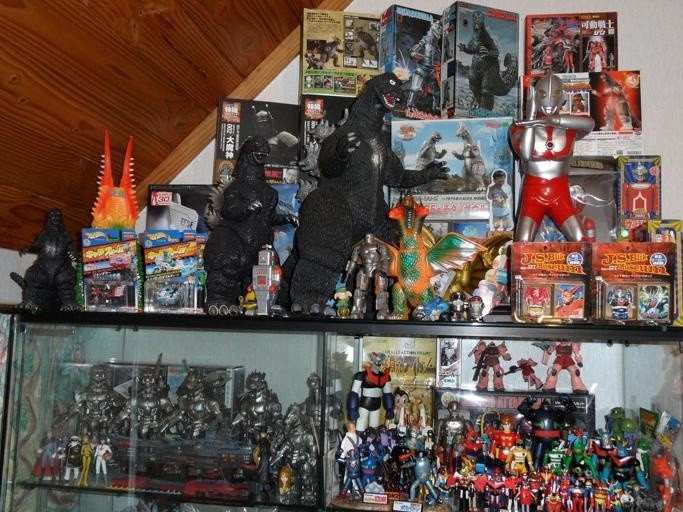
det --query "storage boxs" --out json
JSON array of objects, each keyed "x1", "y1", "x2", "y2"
[
  {"x1": 379, "y1": 4, "x2": 444, "y2": 124},
  {"x1": 212, "y1": 94, "x2": 301, "y2": 186},
  {"x1": 145, "y1": 183, "x2": 215, "y2": 234},
  {"x1": 390, "y1": 116, "x2": 515, "y2": 221},
  {"x1": 299, "y1": 7, "x2": 381, "y2": 98},
  {"x1": 525, "y1": 11, "x2": 617, "y2": 73},
  {"x1": 299, "y1": 94, "x2": 358, "y2": 186},
  {"x1": 523, "y1": 68, "x2": 643, "y2": 160},
  {"x1": 443, "y1": 0, "x2": 520, "y2": 119}
]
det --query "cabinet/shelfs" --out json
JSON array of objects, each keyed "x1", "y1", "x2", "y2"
[{"x1": 0, "y1": 309, "x2": 683, "y2": 512}]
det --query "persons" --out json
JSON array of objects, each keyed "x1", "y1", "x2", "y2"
[
  {"x1": 28, "y1": 352, "x2": 322, "y2": 512},
  {"x1": 333, "y1": 335, "x2": 682, "y2": 512}
]
